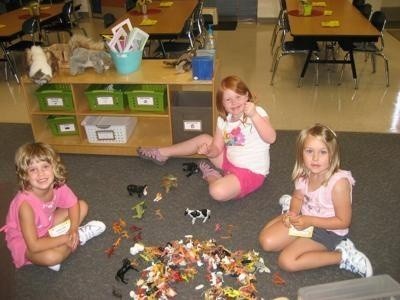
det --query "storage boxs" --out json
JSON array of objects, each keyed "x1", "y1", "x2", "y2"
[
  {"x1": 170, "y1": 91, "x2": 212, "y2": 148},
  {"x1": 296, "y1": 274, "x2": 400, "y2": 300},
  {"x1": 81, "y1": 86, "x2": 127, "y2": 113}
]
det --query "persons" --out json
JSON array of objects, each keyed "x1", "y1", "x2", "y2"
[
  {"x1": 258, "y1": 122, "x2": 373, "y2": 278},
  {"x1": 136, "y1": 76, "x2": 277, "y2": 202},
  {"x1": 0, "y1": 142, "x2": 107, "y2": 272}
]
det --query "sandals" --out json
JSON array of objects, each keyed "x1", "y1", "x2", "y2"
[
  {"x1": 195, "y1": 158, "x2": 223, "y2": 180},
  {"x1": 136, "y1": 146, "x2": 170, "y2": 166}
]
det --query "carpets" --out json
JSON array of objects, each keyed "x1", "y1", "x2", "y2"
[
  {"x1": 212, "y1": 12, "x2": 240, "y2": 32},
  {"x1": 0, "y1": 121, "x2": 400, "y2": 300}
]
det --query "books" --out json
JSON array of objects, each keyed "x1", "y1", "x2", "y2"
[
  {"x1": 191, "y1": 48, "x2": 217, "y2": 81},
  {"x1": 107, "y1": 17, "x2": 150, "y2": 58}
]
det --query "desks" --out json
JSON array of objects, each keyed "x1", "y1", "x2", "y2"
[
  {"x1": 287, "y1": 0, "x2": 385, "y2": 88},
  {"x1": 1, "y1": 1, "x2": 78, "y2": 55},
  {"x1": 97, "y1": 0, "x2": 201, "y2": 60}
]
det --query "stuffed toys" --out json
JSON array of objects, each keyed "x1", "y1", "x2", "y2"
[
  {"x1": 67, "y1": 33, "x2": 112, "y2": 76},
  {"x1": 28, "y1": 45, "x2": 53, "y2": 87},
  {"x1": 162, "y1": 49, "x2": 196, "y2": 74}
]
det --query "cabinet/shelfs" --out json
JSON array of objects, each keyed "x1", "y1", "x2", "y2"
[{"x1": 13, "y1": 55, "x2": 222, "y2": 162}]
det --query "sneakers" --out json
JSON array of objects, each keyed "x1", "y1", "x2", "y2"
[
  {"x1": 49, "y1": 263, "x2": 61, "y2": 272},
  {"x1": 78, "y1": 220, "x2": 106, "y2": 248},
  {"x1": 335, "y1": 237, "x2": 373, "y2": 279},
  {"x1": 278, "y1": 193, "x2": 293, "y2": 214}
]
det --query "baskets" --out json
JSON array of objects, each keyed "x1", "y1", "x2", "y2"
[
  {"x1": 82, "y1": 84, "x2": 131, "y2": 111},
  {"x1": 109, "y1": 50, "x2": 143, "y2": 76}
]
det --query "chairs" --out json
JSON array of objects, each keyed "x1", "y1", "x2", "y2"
[
  {"x1": 37, "y1": 0, "x2": 90, "y2": 50},
  {"x1": 270, "y1": 0, "x2": 288, "y2": 55},
  {"x1": 358, "y1": 3, "x2": 372, "y2": 18},
  {"x1": 101, "y1": 12, "x2": 117, "y2": 28},
  {"x1": 150, "y1": 15, "x2": 200, "y2": 62},
  {"x1": 350, "y1": 1, "x2": 366, "y2": 9},
  {"x1": 335, "y1": 11, "x2": 392, "y2": 89},
  {"x1": 268, "y1": 9, "x2": 322, "y2": 86},
  {"x1": 190, "y1": 1, "x2": 208, "y2": 42},
  {"x1": 123, "y1": 1, "x2": 136, "y2": 12},
  {"x1": 2, "y1": 15, "x2": 45, "y2": 82}
]
